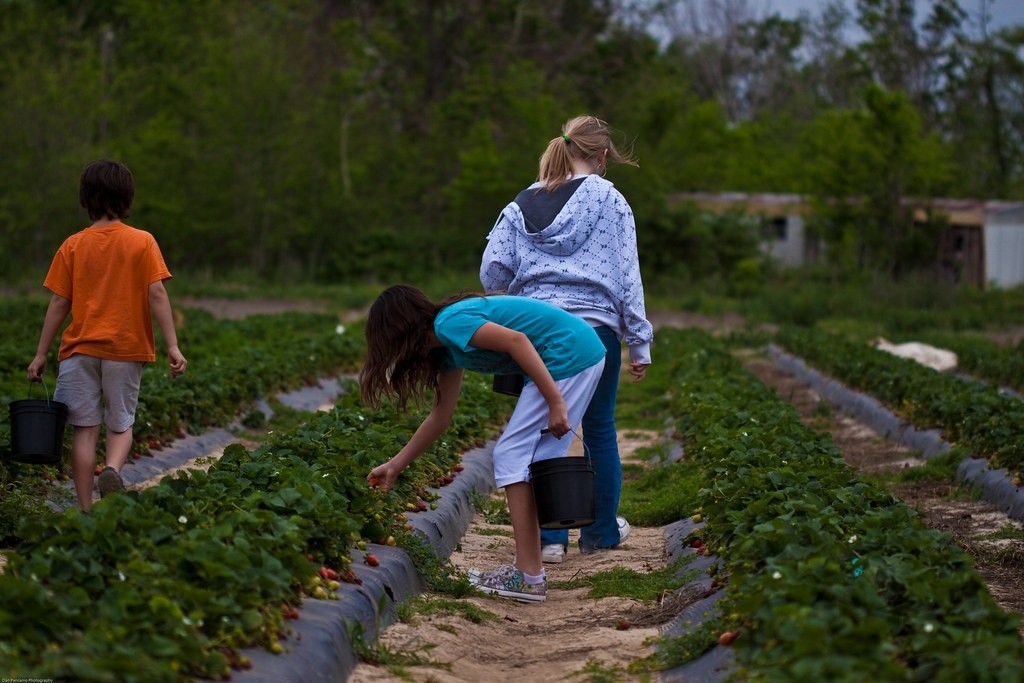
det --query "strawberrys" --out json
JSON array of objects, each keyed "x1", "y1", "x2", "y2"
[
  {"x1": 688, "y1": 505, "x2": 747, "y2": 645},
  {"x1": 971, "y1": 451, "x2": 1024, "y2": 488},
  {"x1": 61, "y1": 433, "x2": 162, "y2": 481},
  {"x1": 210, "y1": 464, "x2": 465, "y2": 679}
]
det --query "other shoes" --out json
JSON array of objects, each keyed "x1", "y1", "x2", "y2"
[
  {"x1": 98, "y1": 467, "x2": 124, "y2": 499},
  {"x1": 615, "y1": 516, "x2": 630, "y2": 541},
  {"x1": 541, "y1": 543, "x2": 563, "y2": 563}
]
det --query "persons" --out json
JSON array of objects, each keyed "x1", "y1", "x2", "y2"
[
  {"x1": 26, "y1": 157, "x2": 186, "y2": 516},
  {"x1": 357, "y1": 285, "x2": 608, "y2": 602},
  {"x1": 477, "y1": 116, "x2": 654, "y2": 563}
]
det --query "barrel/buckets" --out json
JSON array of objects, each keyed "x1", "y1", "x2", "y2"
[{"x1": 528, "y1": 427, "x2": 596, "y2": 530}]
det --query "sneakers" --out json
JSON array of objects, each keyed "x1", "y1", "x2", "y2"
[{"x1": 467, "y1": 563, "x2": 548, "y2": 606}]
